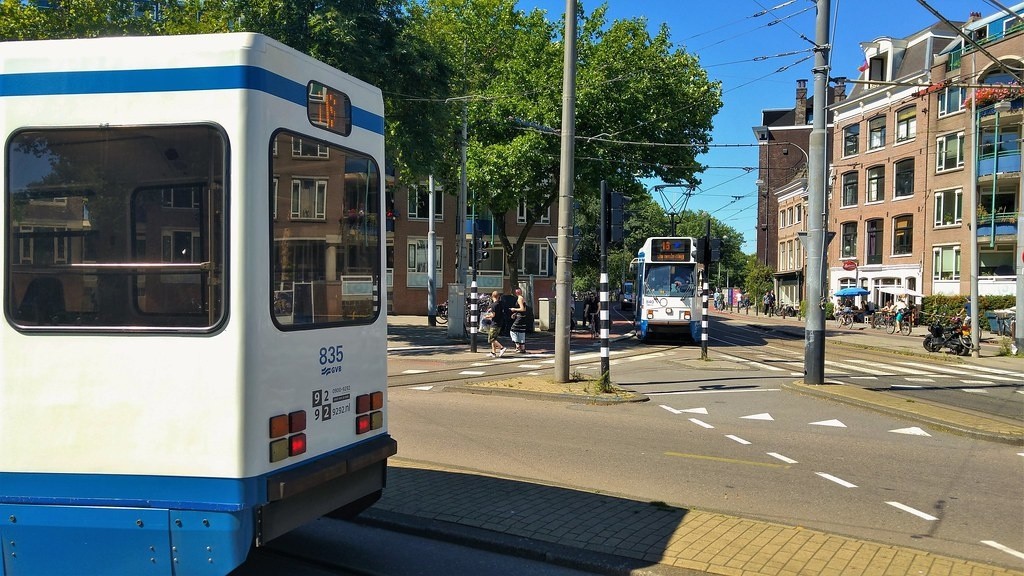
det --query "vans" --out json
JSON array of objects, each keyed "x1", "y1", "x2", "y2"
[{"x1": 620, "y1": 282, "x2": 633, "y2": 311}]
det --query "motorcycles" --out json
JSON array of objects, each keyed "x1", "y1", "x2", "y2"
[{"x1": 922, "y1": 320, "x2": 971, "y2": 356}]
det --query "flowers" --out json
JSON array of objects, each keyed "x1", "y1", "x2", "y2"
[{"x1": 962, "y1": 78, "x2": 1024, "y2": 111}]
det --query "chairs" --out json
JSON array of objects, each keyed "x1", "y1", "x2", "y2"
[{"x1": 832, "y1": 304, "x2": 920, "y2": 327}]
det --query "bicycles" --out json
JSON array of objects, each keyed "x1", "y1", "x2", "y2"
[
  {"x1": 885, "y1": 312, "x2": 912, "y2": 336},
  {"x1": 775, "y1": 299, "x2": 794, "y2": 317},
  {"x1": 955, "y1": 314, "x2": 982, "y2": 341},
  {"x1": 435, "y1": 292, "x2": 534, "y2": 338},
  {"x1": 835, "y1": 306, "x2": 854, "y2": 330},
  {"x1": 585, "y1": 313, "x2": 600, "y2": 341}
]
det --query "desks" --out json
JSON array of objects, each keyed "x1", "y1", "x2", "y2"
[
  {"x1": 852, "y1": 309, "x2": 864, "y2": 323},
  {"x1": 872, "y1": 309, "x2": 896, "y2": 326}
]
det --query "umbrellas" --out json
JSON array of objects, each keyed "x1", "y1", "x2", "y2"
[
  {"x1": 834, "y1": 288, "x2": 869, "y2": 296},
  {"x1": 877, "y1": 285, "x2": 927, "y2": 306}
]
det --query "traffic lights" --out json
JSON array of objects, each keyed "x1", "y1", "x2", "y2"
[
  {"x1": 691, "y1": 238, "x2": 704, "y2": 264},
  {"x1": 608, "y1": 192, "x2": 632, "y2": 243},
  {"x1": 475, "y1": 240, "x2": 490, "y2": 260},
  {"x1": 711, "y1": 238, "x2": 724, "y2": 262}
]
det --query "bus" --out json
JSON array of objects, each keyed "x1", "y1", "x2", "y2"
[
  {"x1": 629, "y1": 183, "x2": 702, "y2": 344},
  {"x1": 0, "y1": 33, "x2": 399, "y2": 575}
]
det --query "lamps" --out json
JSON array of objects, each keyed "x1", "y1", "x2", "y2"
[
  {"x1": 755, "y1": 178, "x2": 765, "y2": 187},
  {"x1": 722, "y1": 234, "x2": 728, "y2": 239},
  {"x1": 702, "y1": 211, "x2": 708, "y2": 216}
]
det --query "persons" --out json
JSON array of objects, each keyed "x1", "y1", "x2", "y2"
[
  {"x1": 486, "y1": 291, "x2": 507, "y2": 359},
  {"x1": 735, "y1": 291, "x2": 749, "y2": 309},
  {"x1": 584, "y1": 292, "x2": 601, "y2": 337},
  {"x1": 714, "y1": 290, "x2": 724, "y2": 312},
  {"x1": 957, "y1": 295, "x2": 971, "y2": 326},
  {"x1": 763, "y1": 290, "x2": 775, "y2": 316},
  {"x1": 570, "y1": 296, "x2": 576, "y2": 330},
  {"x1": 833, "y1": 298, "x2": 870, "y2": 326},
  {"x1": 615, "y1": 288, "x2": 619, "y2": 300},
  {"x1": 882, "y1": 299, "x2": 916, "y2": 333},
  {"x1": 510, "y1": 288, "x2": 528, "y2": 354}
]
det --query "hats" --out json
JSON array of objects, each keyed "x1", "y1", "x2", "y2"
[{"x1": 966, "y1": 297, "x2": 971, "y2": 300}]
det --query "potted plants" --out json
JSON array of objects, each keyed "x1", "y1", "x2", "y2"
[{"x1": 943, "y1": 211, "x2": 953, "y2": 225}]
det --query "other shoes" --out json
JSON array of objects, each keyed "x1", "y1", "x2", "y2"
[
  {"x1": 486, "y1": 352, "x2": 496, "y2": 357},
  {"x1": 499, "y1": 348, "x2": 506, "y2": 357},
  {"x1": 516, "y1": 350, "x2": 526, "y2": 353},
  {"x1": 896, "y1": 329, "x2": 900, "y2": 333}
]
